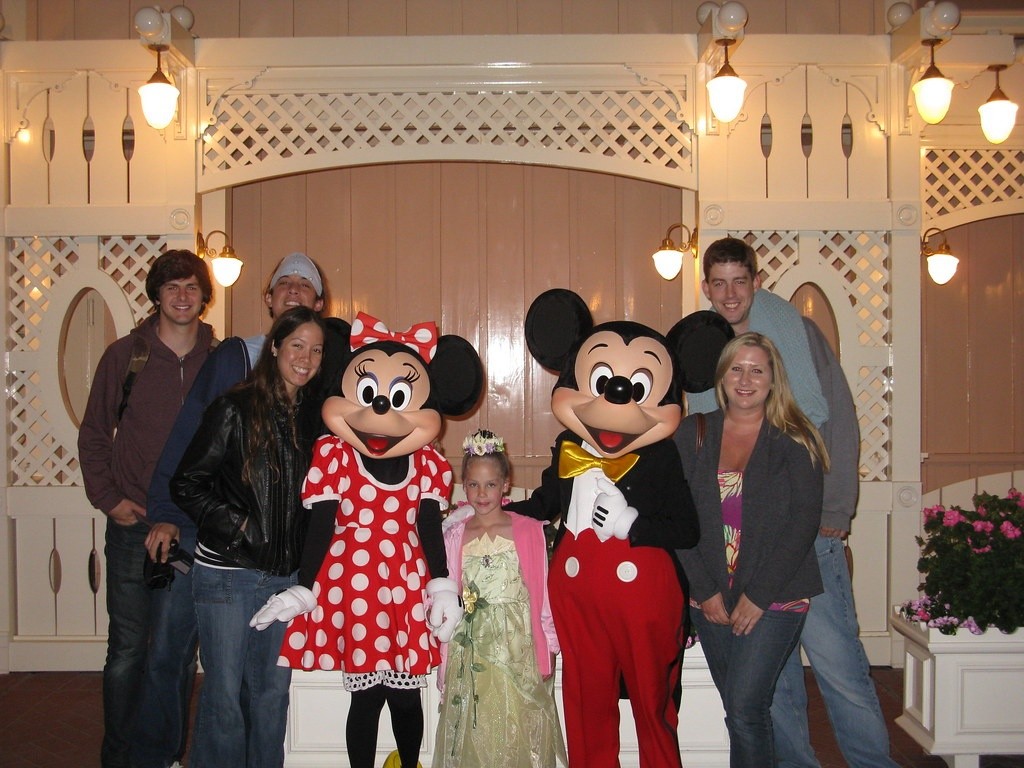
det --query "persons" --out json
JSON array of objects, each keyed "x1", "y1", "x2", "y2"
[
  {"x1": 434, "y1": 429, "x2": 566, "y2": 767},
  {"x1": 673, "y1": 332, "x2": 833, "y2": 767},
  {"x1": 144, "y1": 251, "x2": 327, "y2": 768},
  {"x1": 679, "y1": 236, "x2": 892, "y2": 768},
  {"x1": 167, "y1": 306, "x2": 329, "y2": 768},
  {"x1": 77, "y1": 249, "x2": 222, "y2": 768}
]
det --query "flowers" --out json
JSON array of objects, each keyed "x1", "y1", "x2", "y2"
[
  {"x1": 461, "y1": 583, "x2": 490, "y2": 623},
  {"x1": 900, "y1": 487, "x2": 1024, "y2": 638},
  {"x1": 460, "y1": 434, "x2": 504, "y2": 457}
]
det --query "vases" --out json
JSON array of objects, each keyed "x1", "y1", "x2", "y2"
[{"x1": 892, "y1": 604, "x2": 1024, "y2": 768}]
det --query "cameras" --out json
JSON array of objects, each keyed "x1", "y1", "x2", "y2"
[{"x1": 143, "y1": 539, "x2": 179, "y2": 588}]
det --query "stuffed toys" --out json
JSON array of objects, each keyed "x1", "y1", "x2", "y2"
[
  {"x1": 502, "y1": 288, "x2": 734, "y2": 767},
  {"x1": 249, "y1": 314, "x2": 484, "y2": 768}
]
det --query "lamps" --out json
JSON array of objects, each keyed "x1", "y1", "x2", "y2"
[
  {"x1": 198, "y1": 230, "x2": 245, "y2": 286},
  {"x1": 706, "y1": 39, "x2": 747, "y2": 123},
  {"x1": 979, "y1": 65, "x2": 1020, "y2": 143},
  {"x1": 651, "y1": 223, "x2": 698, "y2": 281},
  {"x1": 918, "y1": 227, "x2": 959, "y2": 285},
  {"x1": 912, "y1": 39, "x2": 956, "y2": 124},
  {"x1": 138, "y1": 45, "x2": 181, "y2": 129}
]
